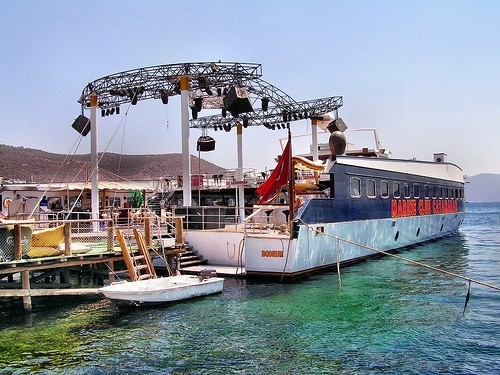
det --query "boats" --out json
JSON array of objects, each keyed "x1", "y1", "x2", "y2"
[
  {"x1": 98, "y1": 272, "x2": 225, "y2": 313},
  {"x1": 175, "y1": 128, "x2": 468, "y2": 282}
]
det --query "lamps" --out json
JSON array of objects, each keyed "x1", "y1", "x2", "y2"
[
  {"x1": 243, "y1": 115, "x2": 248, "y2": 128},
  {"x1": 262, "y1": 98, "x2": 269, "y2": 111},
  {"x1": 102, "y1": 106, "x2": 119, "y2": 117},
  {"x1": 264, "y1": 122, "x2": 290, "y2": 130},
  {"x1": 222, "y1": 86, "x2": 254, "y2": 118},
  {"x1": 197, "y1": 136, "x2": 215, "y2": 151},
  {"x1": 193, "y1": 98, "x2": 203, "y2": 112},
  {"x1": 160, "y1": 90, "x2": 167, "y2": 104},
  {"x1": 71, "y1": 115, "x2": 91, "y2": 136},
  {"x1": 110, "y1": 86, "x2": 144, "y2": 105},
  {"x1": 328, "y1": 118, "x2": 348, "y2": 134},
  {"x1": 214, "y1": 123, "x2": 231, "y2": 132},
  {"x1": 191, "y1": 107, "x2": 197, "y2": 119},
  {"x1": 283, "y1": 112, "x2": 307, "y2": 122},
  {"x1": 199, "y1": 77, "x2": 227, "y2": 97}
]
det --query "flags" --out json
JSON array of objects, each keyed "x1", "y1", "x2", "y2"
[{"x1": 256, "y1": 134, "x2": 292, "y2": 205}]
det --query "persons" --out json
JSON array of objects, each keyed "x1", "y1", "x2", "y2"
[{"x1": 40, "y1": 196, "x2": 48, "y2": 206}]
